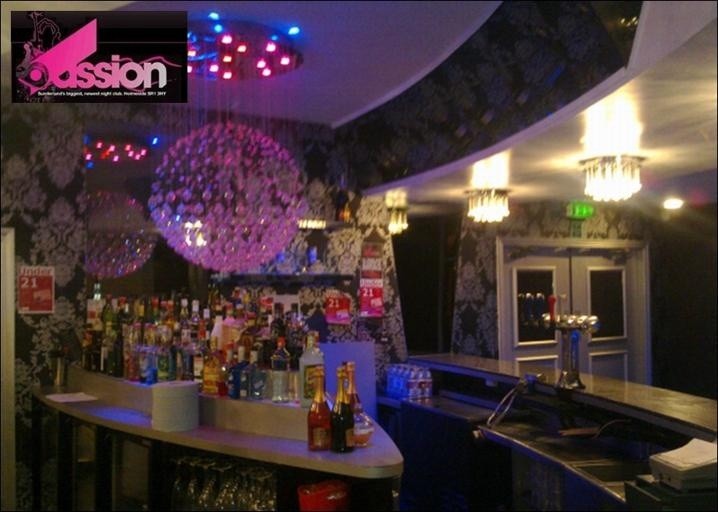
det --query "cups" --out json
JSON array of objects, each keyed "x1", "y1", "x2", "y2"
[{"x1": 169, "y1": 455, "x2": 277, "y2": 512}]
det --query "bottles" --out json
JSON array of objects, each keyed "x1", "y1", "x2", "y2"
[
  {"x1": 283, "y1": 312, "x2": 294, "y2": 344},
  {"x1": 386, "y1": 365, "x2": 396, "y2": 398},
  {"x1": 156, "y1": 331, "x2": 173, "y2": 383},
  {"x1": 81, "y1": 329, "x2": 91, "y2": 369},
  {"x1": 270, "y1": 303, "x2": 282, "y2": 337},
  {"x1": 210, "y1": 312, "x2": 224, "y2": 352},
  {"x1": 193, "y1": 329, "x2": 210, "y2": 379},
  {"x1": 240, "y1": 351, "x2": 267, "y2": 400},
  {"x1": 91, "y1": 333, "x2": 102, "y2": 372},
  {"x1": 227, "y1": 348, "x2": 250, "y2": 398},
  {"x1": 190, "y1": 300, "x2": 200, "y2": 323},
  {"x1": 175, "y1": 329, "x2": 195, "y2": 380},
  {"x1": 158, "y1": 302, "x2": 168, "y2": 330},
  {"x1": 416, "y1": 368, "x2": 425, "y2": 399},
  {"x1": 203, "y1": 306, "x2": 215, "y2": 338},
  {"x1": 106, "y1": 323, "x2": 122, "y2": 376},
  {"x1": 307, "y1": 366, "x2": 333, "y2": 451},
  {"x1": 239, "y1": 312, "x2": 260, "y2": 354},
  {"x1": 179, "y1": 298, "x2": 189, "y2": 321},
  {"x1": 331, "y1": 368, "x2": 355, "y2": 452},
  {"x1": 100, "y1": 327, "x2": 112, "y2": 372},
  {"x1": 407, "y1": 368, "x2": 417, "y2": 398},
  {"x1": 126, "y1": 324, "x2": 142, "y2": 380},
  {"x1": 102, "y1": 295, "x2": 114, "y2": 325},
  {"x1": 399, "y1": 366, "x2": 410, "y2": 399},
  {"x1": 299, "y1": 337, "x2": 323, "y2": 408},
  {"x1": 233, "y1": 306, "x2": 246, "y2": 329},
  {"x1": 423, "y1": 367, "x2": 433, "y2": 399},
  {"x1": 115, "y1": 296, "x2": 129, "y2": 325},
  {"x1": 140, "y1": 324, "x2": 157, "y2": 385},
  {"x1": 149, "y1": 297, "x2": 162, "y2": 327},
  {"x1": 345, "y1": 362, "x2": 360, "y2": 410},
  {"x1": 394, "y1": 365, "x2": 402, "y2": 400},
  {"x1": 166, "y1": 301, "x2": 176, "y2": 329},
  {"x1": 223, "y1": 303, "x2": 235, "y2": 327},
  {"x1": 335, "y1": 174, "x2": 349, "y2": 221},
  {"x1": 270, "y1": 338, "x2": 290, "y2": 404},
  {"x1": 134, "y1": 303, "x2": 147, "y2": 338},
  {"x1": 202, "y1": 337, "x2": 226, "y2": 396},
  {"x1": 90, "y1": 282, "x2": 104, "y2": 332}
]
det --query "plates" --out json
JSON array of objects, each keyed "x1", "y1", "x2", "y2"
[
  {"x1": 46, "y1": 392, "x2": 98, "y2": 402},
  {"x1": 150, "y1": 381, "x2": 199, "y2": 431}
]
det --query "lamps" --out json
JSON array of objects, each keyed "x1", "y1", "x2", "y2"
[
  {"x1": 388, "y1": 206, "x2": 407, "y2": 235},
  {"x1": 578, "y1": 153, "x2": 644, "y2": 201},
  {"x1": 464, "y1": 187, "x2": 511, "y2": 223},
  {"x1": 148, "y1": 9, "x2": 306, "y2": 273},
  {"x1": 71, "y1": 134, "x2": 155, "y2": 280},
  {"x1": 663, "y1": 179, "x2": 685, "y2": 211}
]
